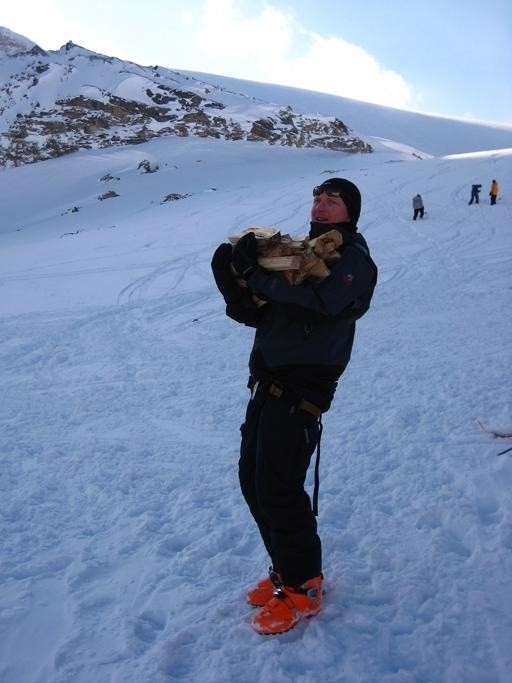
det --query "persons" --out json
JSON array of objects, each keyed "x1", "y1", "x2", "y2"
[
  {"x1": 489, "y1": 180, "x2": 499, "y2": 205},
  {"x1": 469, "y1": 184, "x2": 482, "y2": 204},
  {"x1": 211, "y1": 176, "x2": 377, "y2": 637},
  {"x1": 411, "y1": 194, "x2": 424, "y2": 220}
]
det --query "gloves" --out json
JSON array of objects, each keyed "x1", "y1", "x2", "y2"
[
  {"x1": 211, "y1": 242, "x2": 245, "y2": 303},
  {"x1": 231, "y1": 231, "x2": 258, "y2": 279}
]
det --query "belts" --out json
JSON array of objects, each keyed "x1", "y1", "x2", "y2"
[{"x1": 248, "y1": 377, "x2": 323, "y2": 418}]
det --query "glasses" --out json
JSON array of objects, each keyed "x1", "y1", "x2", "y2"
[{"x1": 312, "y1": 183, "x2": 343, "y2": 198}]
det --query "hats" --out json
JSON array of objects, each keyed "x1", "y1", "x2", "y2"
[{"x1": 321, "y1": 177, "x2": 361, "y2": 221}]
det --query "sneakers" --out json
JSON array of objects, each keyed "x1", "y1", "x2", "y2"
[
  {"x1": 247, "y1": 569, "x2": 282, "y2": 606},
  {"x1": 251, "y1": 575, "x2": 322, "y2": 635}
]
